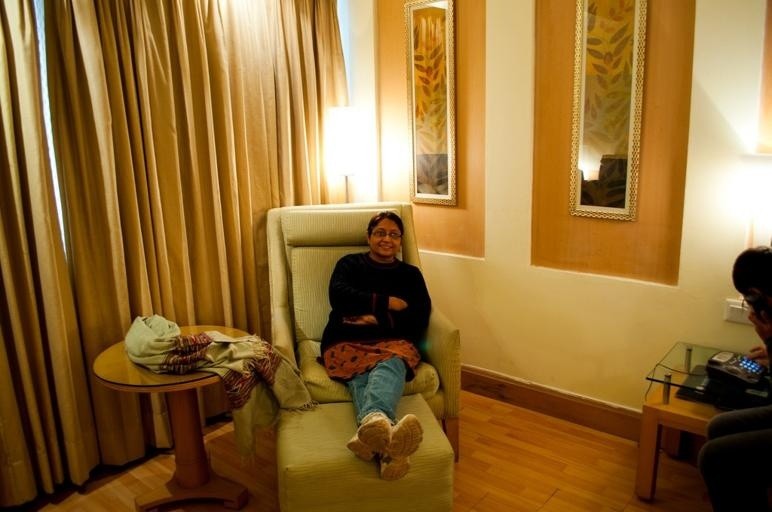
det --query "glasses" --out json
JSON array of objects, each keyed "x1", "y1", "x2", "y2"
[{"x1": 372, "y1": 231, "x2": 401, "y2": 239}]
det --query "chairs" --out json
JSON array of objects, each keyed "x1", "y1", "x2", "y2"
[{"x1": 269, "y1": 202, "x2": 460, "y2": 460}]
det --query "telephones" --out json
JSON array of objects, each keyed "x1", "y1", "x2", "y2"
[{"x1": 705, "y1": 350, "x2": 766, "y2": 385}]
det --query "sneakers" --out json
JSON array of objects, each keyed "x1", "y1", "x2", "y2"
[
  {"x1": 347, "y1": 412, "x2": 391, "y2": 462},
  {"x1": 381, "y1": 414, "x2": 423, "y2": 481}
]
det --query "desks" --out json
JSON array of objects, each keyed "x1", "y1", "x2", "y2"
[{"x1": 636, "y1": 341, "x2": 751, "y2": 500}]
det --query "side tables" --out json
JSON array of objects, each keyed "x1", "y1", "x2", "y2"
[{"x1": 92, "y1": 326, "x2": 253, "y2": 511}]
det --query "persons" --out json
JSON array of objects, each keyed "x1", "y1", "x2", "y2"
[
  {"x1": 320, "y1": 210, "x2": 432, "y2": 482},
  {"x1": 697, "y1": 246, "x2": 772, "y2": 512}
]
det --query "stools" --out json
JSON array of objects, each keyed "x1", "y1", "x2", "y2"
[{"x1": 276, "y1": 394, "x2": 454, "y2": 512}]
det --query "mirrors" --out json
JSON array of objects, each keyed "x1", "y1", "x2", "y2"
[
  {"x1": 569, "y1": 0, "x2": 648, "y2": 225},
  {"x1": 404, "y1": 0, "x2": 455, "y2": 205}
]
